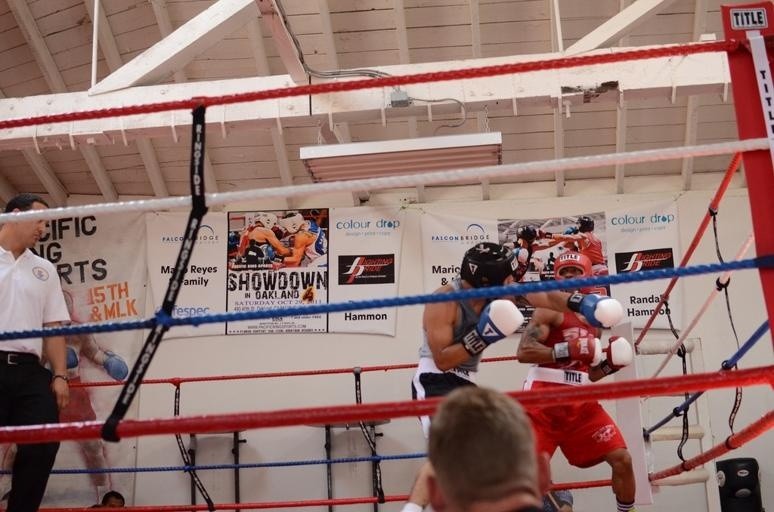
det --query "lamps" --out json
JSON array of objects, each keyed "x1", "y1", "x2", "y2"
[{"x1": 299, "y1": 103, "x2": 504, "y2": 183}]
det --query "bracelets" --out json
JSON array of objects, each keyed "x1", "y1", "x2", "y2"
[{"x1": 49, "y1": 373, "x2": 69, "y2": 384}]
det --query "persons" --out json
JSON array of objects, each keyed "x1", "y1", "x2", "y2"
[
  {"x1": 501, "y1": 224, "x2": 576, "y2": 305},
  {"x1": 38, "y1": 289, "x2": 130, "y2": 508},
  {"x1": 0, "y1": 193, "x2": 70, "y2": 510},
  {"x1": 399, "y1": 241, "x2": 623, "y2": 511},
  {"x1": 234, "y1": 210, "x2": 292, "y2": 266},
  {"x1": 533, "y1": 216, "x2": 603, "y2": 266},
  {"x1": 511, "y1": 249, "x2": 635, "y2": 511},
  {"x1": 268, "y1": 212, "x2": 327, "y2": 272},
  {"x1": 99, "y1": 490, "x2": 127, "y2": 512},
  {"x1": 422, "y1": 385, "x2": 554, "y2": 511}
]
